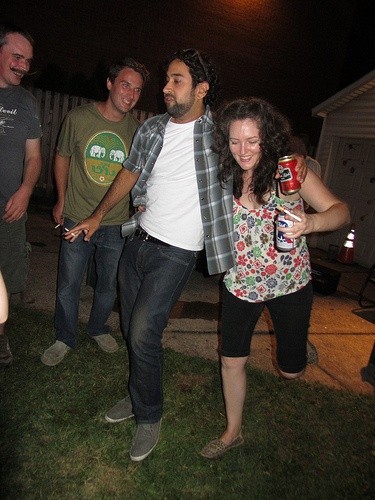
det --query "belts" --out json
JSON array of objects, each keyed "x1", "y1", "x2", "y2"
[{"x1": 137, "y1": 229, "x2": 169, "y2": 248}]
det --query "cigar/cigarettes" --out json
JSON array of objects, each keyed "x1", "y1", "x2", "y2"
[
  {"x1": 53, "y1": 224, "x2": 60, "y2": 229},
  {"x1": 277, "y1": 205, "x2": 301, "y2": 222},
  {"x1": 65, "y1": 228, "x2": 75, "y2": 236}
]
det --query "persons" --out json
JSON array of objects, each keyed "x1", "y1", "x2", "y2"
[
  {"x1": 200, "y1": 94, "x2": 350, "y2": 459},
  {"x1": 40, "y1": 57, "x2": 149, "y2": 366},
  {"x1": 0, "y1": 30, "x2": 41, "y2": 365},
  {"x1": 68, "y1": 48, "x2": 219, "y2": 461}
]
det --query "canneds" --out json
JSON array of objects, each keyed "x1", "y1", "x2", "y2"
[
  {"x1": 278, "y1": 156, "x2": 301, "y2": 195},
  {"x1": 275, "y1": 213, "x2": 294, "y2": 252}
]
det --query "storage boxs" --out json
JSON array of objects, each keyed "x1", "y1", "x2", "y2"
[{"x1": 310, "y1": 263, "x2": 341, "y2": 296}]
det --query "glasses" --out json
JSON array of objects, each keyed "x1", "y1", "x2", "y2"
[{"x1": 167, "y1": 48, "x2": 211, "y2": 83}]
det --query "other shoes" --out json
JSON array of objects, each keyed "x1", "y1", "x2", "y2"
[
  {"x1": 87, "y1": 332, "x2": 119, "y2": 354},
  {"x1": 105, "y1": 394, "x2": 135, "y2": 423},
  {"x1": 0, "y1": 335, "x2": 13, "y2": 367},
  {"x1": 201, "y1": 435, "x2": 245, "y2": 458},
  {"x1": 307, "y1": 340, "x2": 318, "y2": 365},
  {"x1": 129, "y1": 417, "x2": 162, "y2": 461},
  {"x1": 40, "y1": 340, "x2": 74, "y2": 366}
]
